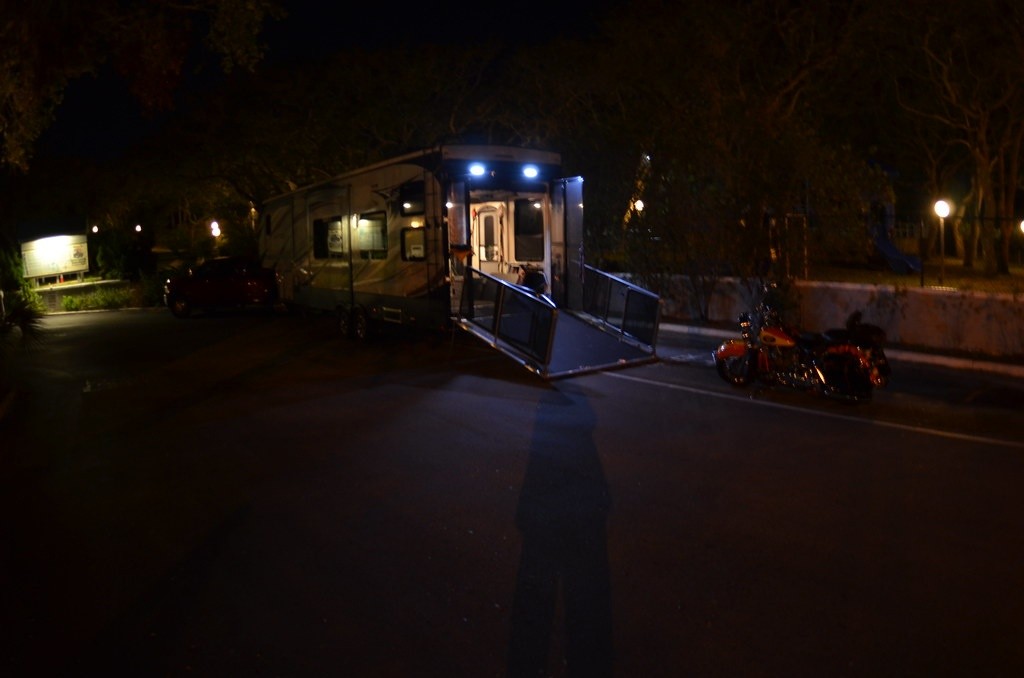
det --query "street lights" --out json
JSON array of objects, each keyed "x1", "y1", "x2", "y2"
[{"x1": 932, "y1": 199, "x2": 955, "y2": 289}]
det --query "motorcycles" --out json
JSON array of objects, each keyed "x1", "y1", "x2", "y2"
[{"x1": 710, "y1": 282, "x2": 884, "y2": 410}]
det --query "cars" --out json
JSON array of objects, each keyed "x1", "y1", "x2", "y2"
[{"x1": 163, "y1": 257, "x2": 287, "y2": 316}]
